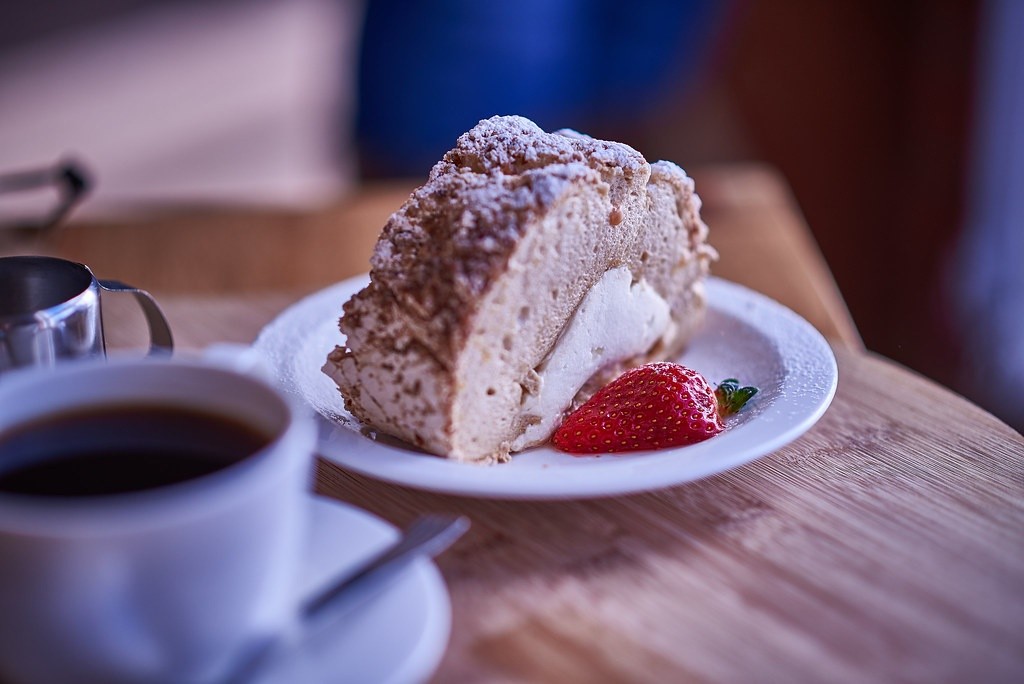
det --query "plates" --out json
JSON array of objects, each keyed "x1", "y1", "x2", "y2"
[
  {"x1": 252, "y1": 273, "x2": 836, "y2": 500},
  {"x1": 213, "y1": 486, "x2": 451, "y2": 683}
]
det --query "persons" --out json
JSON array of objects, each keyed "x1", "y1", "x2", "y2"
[{"x1": 667, "y1": 0, "x2": 1024, "y2": 443}]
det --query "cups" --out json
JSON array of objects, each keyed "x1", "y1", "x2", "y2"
[
  {"x1": 0, "y1": 254, "x2": 174, "y2": 365},
  {"x1": 0, "y1": 354, "x2": 315, "y2": 684}
]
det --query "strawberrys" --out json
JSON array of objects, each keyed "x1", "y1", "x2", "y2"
[{"x1": 552, "y1": 363, "x2": 724, "y2": 455}]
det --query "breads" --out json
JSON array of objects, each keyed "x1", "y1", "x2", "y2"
[{"x1": 320, "y1": 116, "x2": 718, "y2": 461}]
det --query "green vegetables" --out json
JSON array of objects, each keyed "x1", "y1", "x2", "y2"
[{"x1": 716, "y1": 379, "x2": 759, "y2": 412}]
